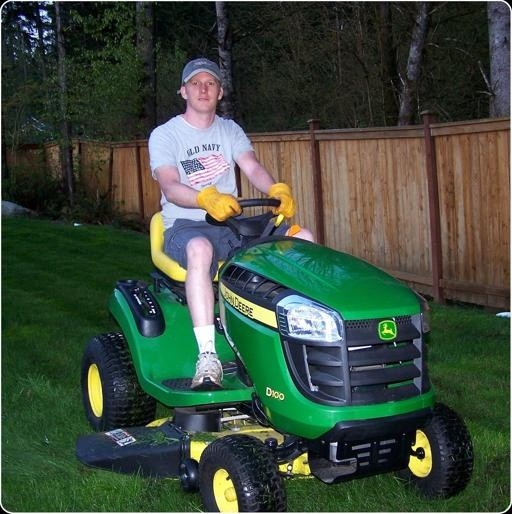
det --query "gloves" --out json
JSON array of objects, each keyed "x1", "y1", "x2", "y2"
[
  {"x1": 196, "y1": 186, "x2": 242, "y2": 224},
  {"x1": 266, "y1": 182, "x2": 297, "y2": 219}
]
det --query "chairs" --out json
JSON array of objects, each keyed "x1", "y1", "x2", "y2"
[{"x1": 149, "y1": 208, "x2": 236, "y2": 284}]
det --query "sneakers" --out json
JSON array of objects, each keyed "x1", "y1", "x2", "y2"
[{"x1": 190, "y1": 351, "x2": 224, "y2": 389}]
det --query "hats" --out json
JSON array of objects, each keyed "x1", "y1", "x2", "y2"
[{"x1": 181, "y1": 59, "x2": 223, "y2": 84}]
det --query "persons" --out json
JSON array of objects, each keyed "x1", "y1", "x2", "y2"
[{"x1": 145, "y1": 58, "x2": 318, "y2": 393}]
